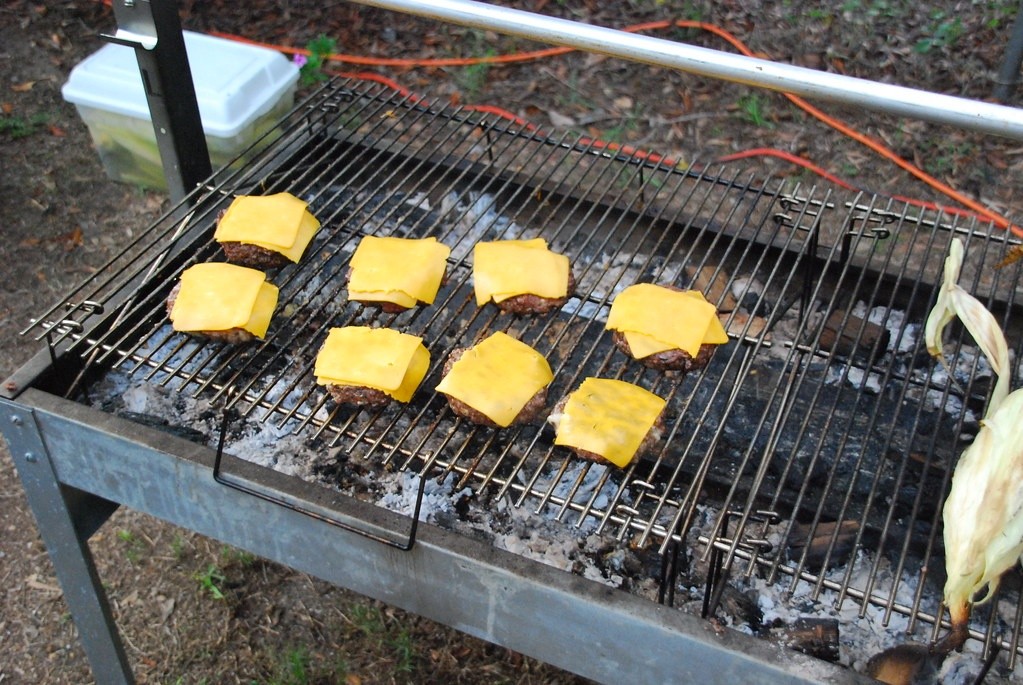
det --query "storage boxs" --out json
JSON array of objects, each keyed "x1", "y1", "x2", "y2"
[{"x1": 59, "y1": 30, "x2": 300, "y2": 196}]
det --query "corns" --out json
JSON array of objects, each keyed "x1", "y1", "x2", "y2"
[{"x1": 944, "y1": 386, "x2": 1023, "y2": 647}]
